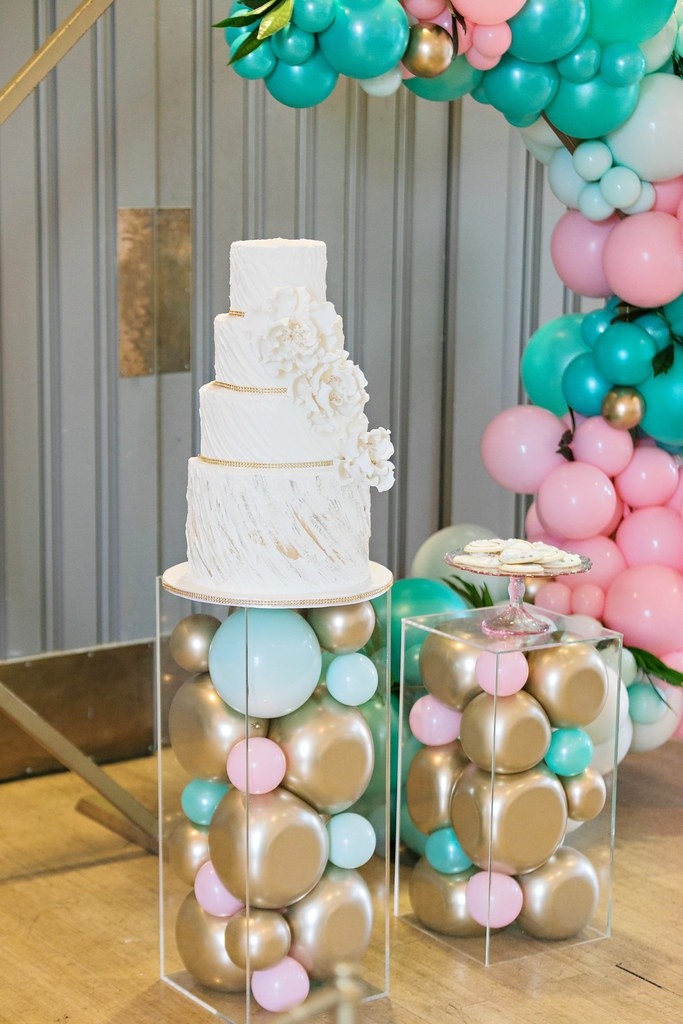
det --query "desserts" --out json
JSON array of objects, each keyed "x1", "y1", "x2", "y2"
[{"x1": 453, "y1": 539, "x2": 584, "y2": 572}]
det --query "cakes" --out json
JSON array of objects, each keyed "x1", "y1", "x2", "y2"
[{"x1": 185, "y1": 237, "x2": 396, "y2": 590}]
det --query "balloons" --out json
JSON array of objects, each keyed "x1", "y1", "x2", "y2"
[{"x1": 209, "y1": 0, "x2": 682, "y2": 752}]
